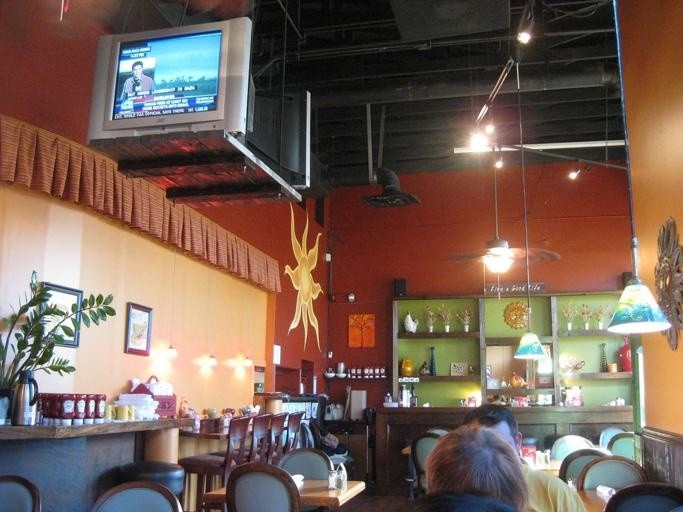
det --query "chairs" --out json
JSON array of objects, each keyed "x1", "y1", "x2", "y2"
[
  {"x1": 88, "y1": 479, "x2": 184, "y2": 512},
  {"x1": 0, "y1": 473, "x2": 40, "y2": 510},
  {"x1": 404, "y1": 427, "x2": 682, "y2": 512},
  {"x1": 176, "y1": 409, "x2": 352, "y2": 511}
]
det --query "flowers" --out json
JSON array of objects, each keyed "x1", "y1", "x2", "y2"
[
  {"x1": 424, "y1": 304, "x2": 435, "y2": 327},
  {"x1": 454, "y1": 303, "x2": 472, "y2": 325},
  {"x1": 436, "y1": 302, "x2": 453, "y2": 325}
]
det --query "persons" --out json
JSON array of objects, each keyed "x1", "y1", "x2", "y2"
[
  {"x1": 464, "y1": 404, "x2": 586, "y2": 512},
  {"x1": 120, "y1": 61, "x2": 152, "y2": 100},
  {"x1": 425, "y1": 423, "x2": 529, "y2": 512},
  {"x1": 396, "y1": 490, "x2": 518, "y2": 512}
]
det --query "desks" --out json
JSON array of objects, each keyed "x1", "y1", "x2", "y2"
[{"x1": 200, "y1": 479, "x2": 365, "y2": 508}]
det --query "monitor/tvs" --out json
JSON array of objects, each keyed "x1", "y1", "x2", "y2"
[
  {"x1": 246, "y1": 90, "x2": 312, "y2": 189},
  {"x1": 87, "y1": 17, "x2": 255, "y2": 146}
]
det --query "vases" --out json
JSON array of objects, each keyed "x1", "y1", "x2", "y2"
[
  {"x1": 462, "y1": 323, "x2": 469, "y2": 331},
  {"x1": 443, "y1": 324, "x2": 450, "y2": 333},
  {"x1": 427, "y1": 325, "x2": 433, "y2": 332}
]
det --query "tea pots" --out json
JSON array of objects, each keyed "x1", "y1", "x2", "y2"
[{"x1": 11, "y1": 370, "x2": 40, "y2": 427}]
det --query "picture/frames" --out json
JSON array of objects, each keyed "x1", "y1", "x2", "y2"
[
  {"x1": 39, "y1": 282, "x2": 83, "y2": 348},
  {"x1": 124, "y1": 300, "x2": 152, "y2": 357}
]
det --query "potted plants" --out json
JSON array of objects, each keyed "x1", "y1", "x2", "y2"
[{"x1": 0, "y1": 282, "x2": 117, "y2": 425}]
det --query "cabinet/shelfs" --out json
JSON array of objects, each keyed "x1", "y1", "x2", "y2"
[
  {"x1": 552, "y1": 288, "x2": 640, "y2": 412},
  {"x1": 394, "y1": 297, "x2": 481, "y2": 403},
  {"x1": 480, "y1": 292, "x2": 556, "y2": 410}
]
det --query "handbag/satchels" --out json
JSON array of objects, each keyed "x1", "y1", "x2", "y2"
[{"x1": 323, "y1": 433, "x2": 339, "y2": 449}]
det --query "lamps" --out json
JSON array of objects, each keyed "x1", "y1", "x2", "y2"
[
  {"x1": 512, "y1": 305, "x2": 549, "y2": 359},
  {"x1": 606, "y1": 235, "x2": 671, "y2": 341},
  {"x1": 516, "y1": 3, "x2": 540, "y2": 44},
  {"x1": 446, "y1": 235, "x2": 559, "y2": 276},
  {"x1": 328, "y1": 292, "x2": 355, "y2": 303},
  {"x1": 567, "y1": 167, "x2": 587, "y2": 183}
]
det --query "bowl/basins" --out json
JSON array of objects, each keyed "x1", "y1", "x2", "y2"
[{"x1": 324, "y1": 372, "x2": 347, "y2": 379}]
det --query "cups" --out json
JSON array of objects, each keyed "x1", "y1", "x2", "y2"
[
  {"x1": 608, "y1": 363, "x2": 617, "y2": 373},
  {"x1": 106, "y1": 403, "x2": 137, "y2": 421}
]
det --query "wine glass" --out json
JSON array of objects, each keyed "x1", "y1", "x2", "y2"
[{"x1": 500, "y1": 375, "x2": 511, "y2": 388}]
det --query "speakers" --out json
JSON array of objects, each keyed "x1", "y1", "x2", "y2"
[
  {"x1": 394, "y1": 278, "x2": 406, "y2": 297},
  {"x1": 622, "y1": 272, "x2": 632, "y2": 290}
]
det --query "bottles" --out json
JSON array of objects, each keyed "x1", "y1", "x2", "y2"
[
  {"x1": 543, "y1": 447, "x2": 550, "y2": 463},
  {"x1": 399, "y1": 356, "x2": 416, "y2": 377},
  {"x1": 37, "y1": 392, "x2": 107, "y2": 426},
  {"x1": 617, "y1": 336, "x2": 632, "y2": 371},
  {"x1": 399, "y1": 385, "x2": 408, "y2": 407},
  {"x1": 383, "y1": 392, "x2": 392, "y2": 402},
  {"x1": 256, "y1": 383, "x2": 264, "y2": 393},
  {"x1": 596, "y1": 485, "x2": 616, "y2": 497},
  {"x1": 327, "y1": 470, "x2": 337, "y2": 489},
  {"x1": 337, "y1": 469, "x2": 348, "y2": 492},
  {"x1": 351, "y1": 366, "x2": 386, "y2": 378}
]
